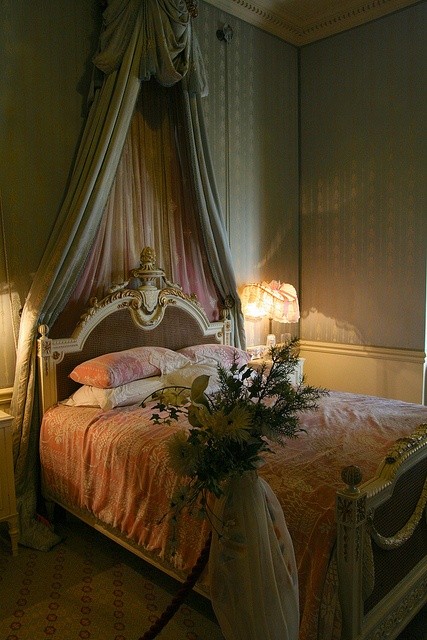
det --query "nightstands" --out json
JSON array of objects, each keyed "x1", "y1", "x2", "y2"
[
  {"x1": 0, "y1": 410, "x2": 20, "y2": 558},
  {"x1": 249, "y1": 355, "x2": 305, "y2": 386}
]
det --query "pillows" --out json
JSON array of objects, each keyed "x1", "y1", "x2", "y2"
[
  {"x1": 68, "y1": 346, "x2": 191, "y2": 389},
  {"x1": 170, "y1": 363, "x2": 267, "y2": 395},
  {"x1": 177, "y1": 343, "x2": 252, "y2": 369},
  {"x1": 64, "y1": 375, "x2": 168, "y2": 410}
]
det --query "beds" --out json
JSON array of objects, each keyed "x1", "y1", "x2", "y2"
[{"x1": 34, "y1": 245, "x2": 427, "y2": 640}]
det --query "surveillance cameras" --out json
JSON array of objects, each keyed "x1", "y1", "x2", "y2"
[{"x1": 215, "y1": 25, "x2": 231, "y2": 42}]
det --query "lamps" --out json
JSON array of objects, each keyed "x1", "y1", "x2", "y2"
[{"x1": 241, "y1": 281, "x2": 301, "y2": 334}]
